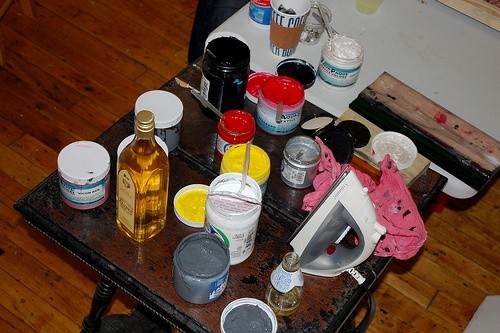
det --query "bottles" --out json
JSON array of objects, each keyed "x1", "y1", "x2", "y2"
[
  {"x1": 265, "y1": 252, "x2": 305, "y2": 316},
  {"x1": 114, "y1": 110, "x2": 169, "y2": 241}
]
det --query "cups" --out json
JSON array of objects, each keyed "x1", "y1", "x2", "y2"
[
  {"x1": 116, "y1": 132, "x2": 169, "y2": 169},
  {"x1": 356, "y1": 0, "x2": 384, "y2": 15},
  {"x1": 270, "y1": 0, "x2": 311, "y2": 56},
  {"x1": 298, "y1": 2, "x2": 332, "y2": 45}
]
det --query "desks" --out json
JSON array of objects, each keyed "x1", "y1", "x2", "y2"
[
  {"x1": 12, "y1": 56, "x2": 449, "y2": 333},
  {"x1": 188, "y1": 0, "x2": 500, "y2": 211}
]
